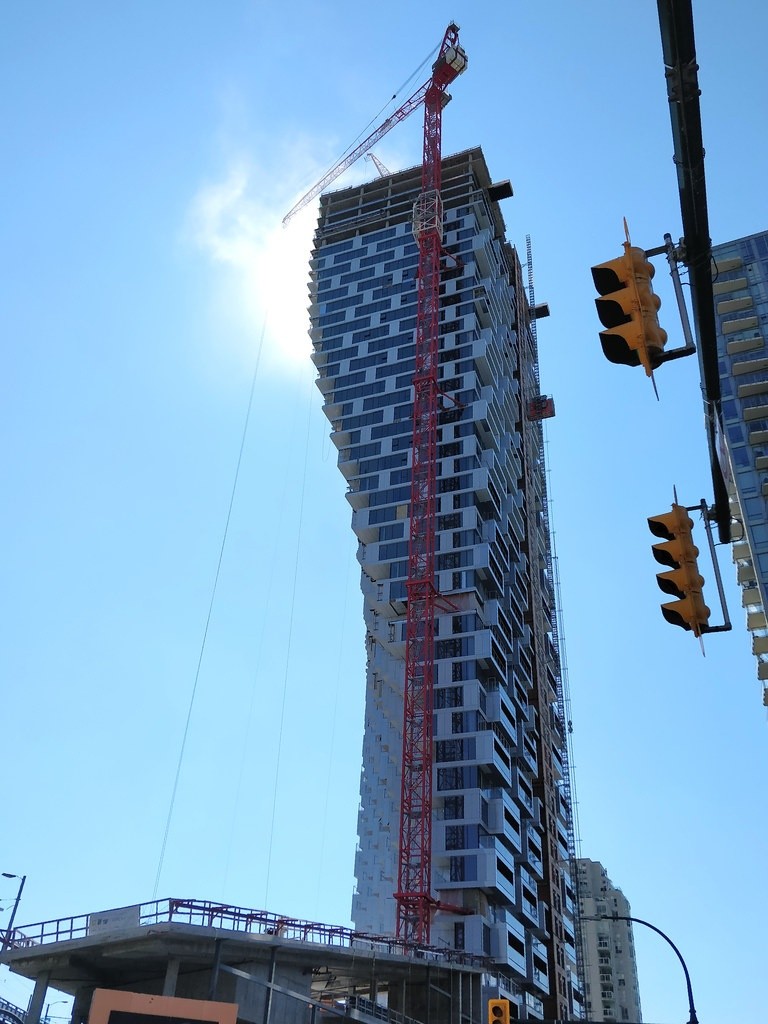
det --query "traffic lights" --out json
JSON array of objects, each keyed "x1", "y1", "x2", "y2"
[
  {"x1": 488, "y1": 999, "x2": 509, "y2": 1024},
  {"x1": 590, "y1": 242, "x2": 667, "y2": 379},
  {"x1": 642, "y1": 500, "x2": 712, "y2": 639}
]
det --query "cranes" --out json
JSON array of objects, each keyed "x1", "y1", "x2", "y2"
[{"x1": 283, "y1": 20, "x2": 469, "y2": 958}]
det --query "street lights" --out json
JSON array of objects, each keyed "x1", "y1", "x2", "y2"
[
  {"x1": 0, "y1": 873, "x2": 26, "y2": 966},
  {"x1": 578, "y1": 914, "x2": 699, "y2": 1024}
]
